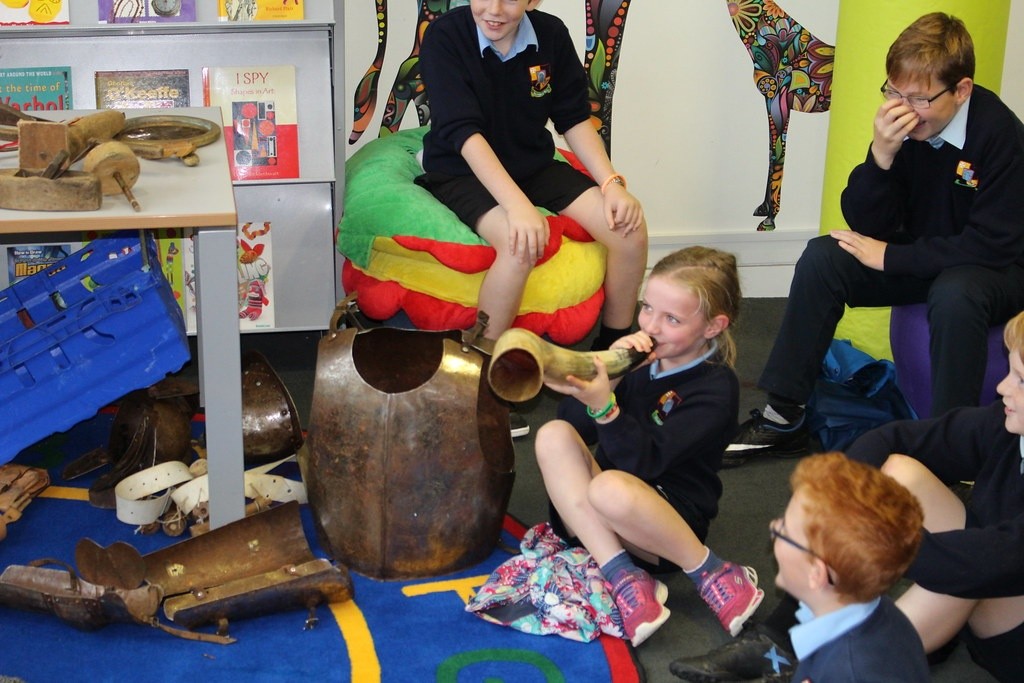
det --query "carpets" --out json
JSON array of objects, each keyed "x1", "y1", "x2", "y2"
[{"x1": 1, "y1": 347, "x2": 647, "y2": 682}]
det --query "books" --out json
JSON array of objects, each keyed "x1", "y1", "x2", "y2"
[
  {"x1": 5, "y1": 245, "x2": 72, "y2": 287},
  {"x1": 81, "y1": 228, "x2": 183, "y2": 312},
  {"x1": 99, "y1": 0, "x2": 196, "y2": 25},
  {"x1": 202, "y1": 66, "x2": 299, "y2": 178},
  {"x1": 93, "y1": 69, "x2": 190, "y2": 108},
  {"x1": 1, "y1": 1, "x2": 70, "y2": 26},
  {"x1": 218, "y1": 0, "x2": 302, "y2": 21},
  {"x1": 183, "y1": 222, "x2": 275, "y2": 331},
  {"x1": 0, "y1": 67, "x2": 73, "y2": 110}
]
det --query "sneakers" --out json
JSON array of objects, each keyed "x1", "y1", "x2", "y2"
[
  {"x1": 607, "y1": 570, "x2": 669, "y2": 646},
  {"x1": 720, "y1": 411, "x2": 811, "y2": 469},
  {"x1": 670, "y1": 631, "x2": 800, "y2": 682},
  {"x1": 702, "y1": 563, "x2": 764, "y2": 638}
]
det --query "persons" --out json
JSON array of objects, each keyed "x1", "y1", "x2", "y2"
[
  {"x1": 536, "y1": 246, "x2": 767, "y2": 646},
  {"x1": 669, "y1": 310, "x2": 1024, "y2": 683},
  {"x1": 720, "y1": 12, "x2": 1024, "y2": 467},
  {"x1": 770, "y1": 451, "x2": 932, "y2": 683},
  {"x1": 419, "y1": 1, "x2": 649, "y2": 438}
]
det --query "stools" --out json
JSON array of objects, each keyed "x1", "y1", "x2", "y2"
[
  {"x1": 339, "y1": 126, "x2": 616, "y2": 351},
  {"x1": 880, "y1": 301, "x2": 1015, "y2": 429}
]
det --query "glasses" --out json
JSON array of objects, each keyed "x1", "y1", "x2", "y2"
[
  {"x1": 880, "y1": 78, "x2": 955, "y2": 109},
  {"x1": 768, "y1": 516, "x2": 829, "y2": 562}
]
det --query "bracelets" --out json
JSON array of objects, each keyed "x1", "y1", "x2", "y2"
[
  {"x1": 586, "y1": 391, "x2": 619, "y2": 421},
  {"x1": 602, "y1": 174, "x2": 626, "y2": 197}
]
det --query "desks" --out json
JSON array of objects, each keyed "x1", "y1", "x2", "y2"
[{"x1": 3, "y1": 104, "x2": 243, "y2": 540}]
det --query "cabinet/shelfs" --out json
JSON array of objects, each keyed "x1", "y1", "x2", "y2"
[{"x1": 0, "y1": 3, "x2": 358, "y2": 357}]
